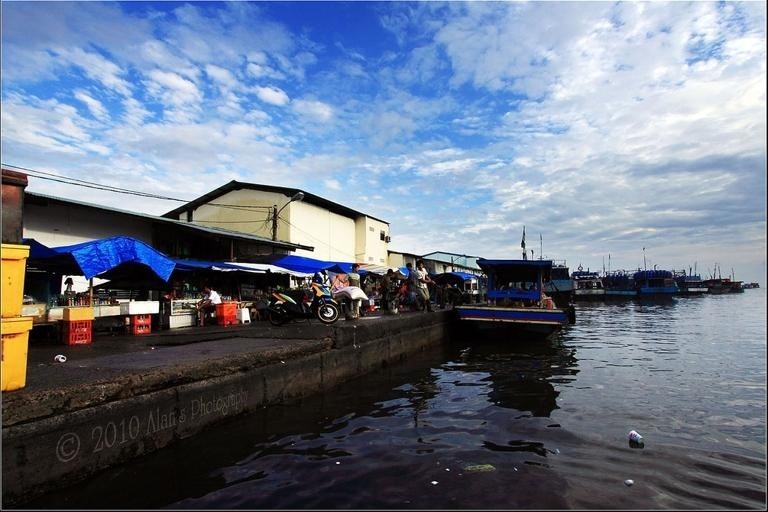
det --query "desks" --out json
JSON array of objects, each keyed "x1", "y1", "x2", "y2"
[{"x1": 47, "y1": 305, "x2": 120, "y2": 344}]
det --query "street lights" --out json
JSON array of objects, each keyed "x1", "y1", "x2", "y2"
[{"x1": 272, "y1": 191, "x2": 305, "y2": 241}]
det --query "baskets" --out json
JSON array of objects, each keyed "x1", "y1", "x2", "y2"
[
  {"x1": 63, "y1": 320, "x2": 92, "y2": 345},
  {"x1": 216, "y1": 303, "x2": 238, "y2": 326},
  {"x1": 131, "y1": 314, "x2": 152, "y2": 336}
]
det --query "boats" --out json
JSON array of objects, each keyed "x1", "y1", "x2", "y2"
[
  {"x1": 454, "y1": 224, "x2": 569, "y2": 342},
  {"x1": 512, "y1": 234, "x2": 572, "y2": 307},
  {"x1": 572, "y1": 246, "x2": 761, "y2": 298}
]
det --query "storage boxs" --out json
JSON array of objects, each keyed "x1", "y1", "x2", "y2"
[
  {"x1": 120, "y1": 301, "x2": 160, "y2": 315},
  {"x1": 63, "y1": 306, "x2": 95, "y2": 320},
  {"x1": 0, "y1": 244, "x2": 30, "y2": 318},
  {"x1": 0, "y1": 169, "x2": 28, "y2": 244},
  {"x1": 0, "y1": 317, "x2": 33, "y2": 391}
]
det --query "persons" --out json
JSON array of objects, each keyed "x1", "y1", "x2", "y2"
[
  {"x1": 500, "y1": 281, "x2": 537, "y2": 306},
  {"x1": 198, "y1": 285, "x2": 222, "y2": 327},
  {"x1": 336, "y1": 261, "x2": 434, "y2": 316}
]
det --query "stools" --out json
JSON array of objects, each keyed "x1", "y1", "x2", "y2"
[{"x1": 236, "y1": 308, "x2": 251, "y2": 324}]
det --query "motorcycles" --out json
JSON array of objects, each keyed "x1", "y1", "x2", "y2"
[
  {"x1": 263, "y1": 271, "x2": 341, "y2": 326},
  {"x1": 386, "y1": 279, "x2": 425, "y2": 314}
]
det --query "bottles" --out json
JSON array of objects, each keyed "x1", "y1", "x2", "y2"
[
  {"x1": 137, "y1": 315, "x2": 148, "y2": 319},
  {"x1": 46, "y1": 297, "x2": 111, "y2": 308},
  {"x1": 137, "y1": 325, "x2": 148, "y2": 330}
]
